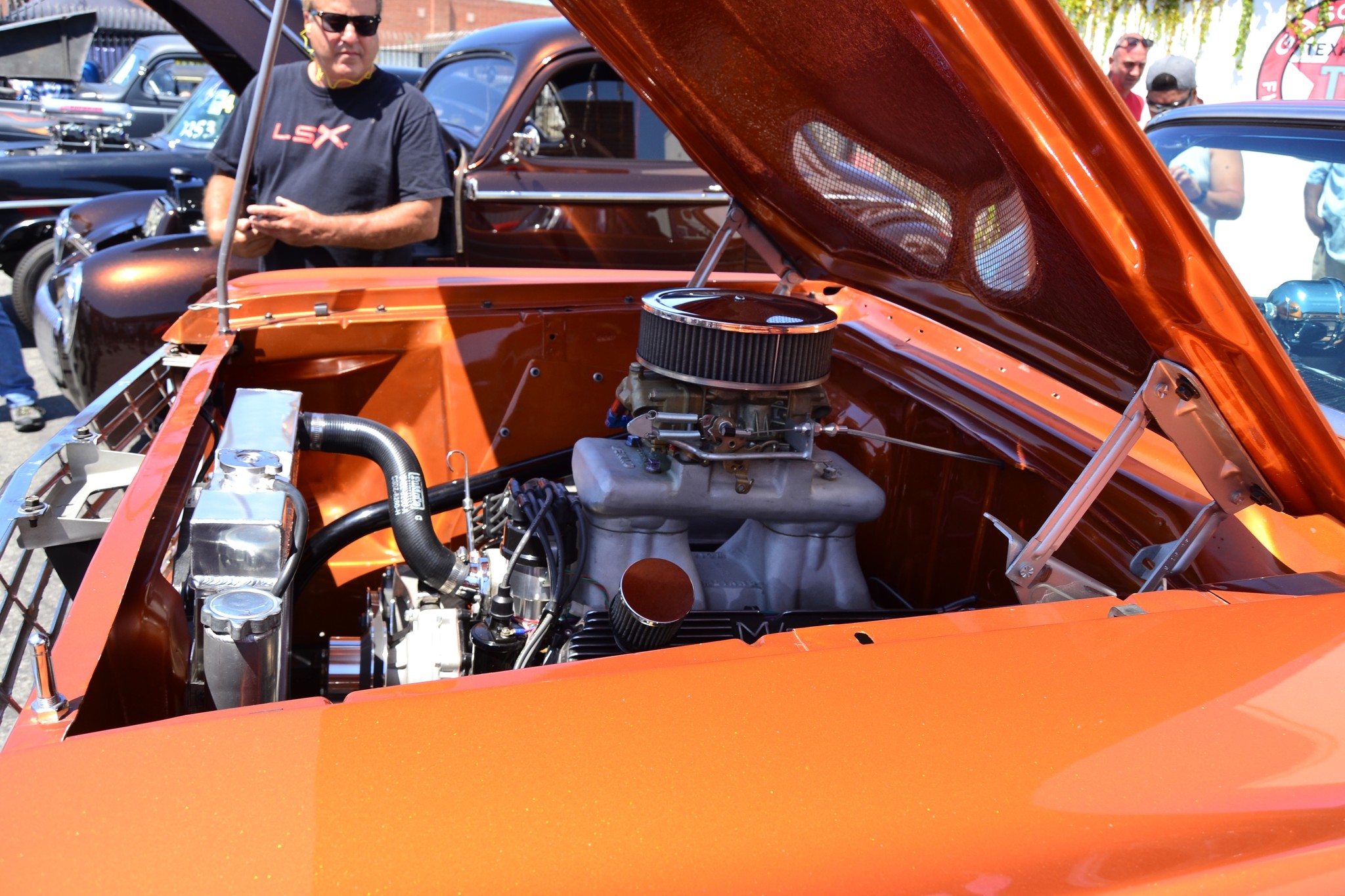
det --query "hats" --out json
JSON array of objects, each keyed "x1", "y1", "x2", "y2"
[{"x1": 1146, "y1": 56, "x2": 1197, "y2": 91}]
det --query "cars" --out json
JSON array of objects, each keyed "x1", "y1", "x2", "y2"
[
  {"x1": 1137, "y1": 106, "x2": 1344, "y2": 424},
  {"x1": 1, "y1": 0, "x2": 1345, "y2": 894},
  {"x1": 0, "y1": 11, "x2": 955, "y2": 427}
]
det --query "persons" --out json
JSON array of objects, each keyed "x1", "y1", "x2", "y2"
[
  {"x1": 1145, "y1": 56, "x2": 1244, "y2": 241},
  {"x1": 1106, "y1": 33, "x2": 1148, "y2": 122},
  {"x1": 201, "y1": 0, "x2": 454, "y2": 274},
  {"x1": 0, "y1": 305, "x2": 48, "y2": 432},
  {"x1": 1303, "y1": 161, "x2": 1345, "y2": 286}
]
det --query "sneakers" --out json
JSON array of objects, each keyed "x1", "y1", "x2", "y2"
[{"x1": 10, "y1": 402, "x2": 46, "y2": 431}]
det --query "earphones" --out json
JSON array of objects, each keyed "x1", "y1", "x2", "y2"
[{"x1": 304, "y1": 23, "x2": 313, "y2": 33}]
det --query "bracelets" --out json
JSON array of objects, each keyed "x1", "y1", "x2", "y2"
[{"x1": 1189, "y1": 185, "x2": 1207, "y2": 205}]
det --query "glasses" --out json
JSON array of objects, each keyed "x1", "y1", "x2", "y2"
[
  {"x1": 1115, "y1": 36, "x2": 1154, "y2": 50},
  {"x1": 1146, "y1": 89, "x2": 1194, "y2": 113},
  {"x1": 311, "y1": 10, "x2": 382, "y2": 36}
]
